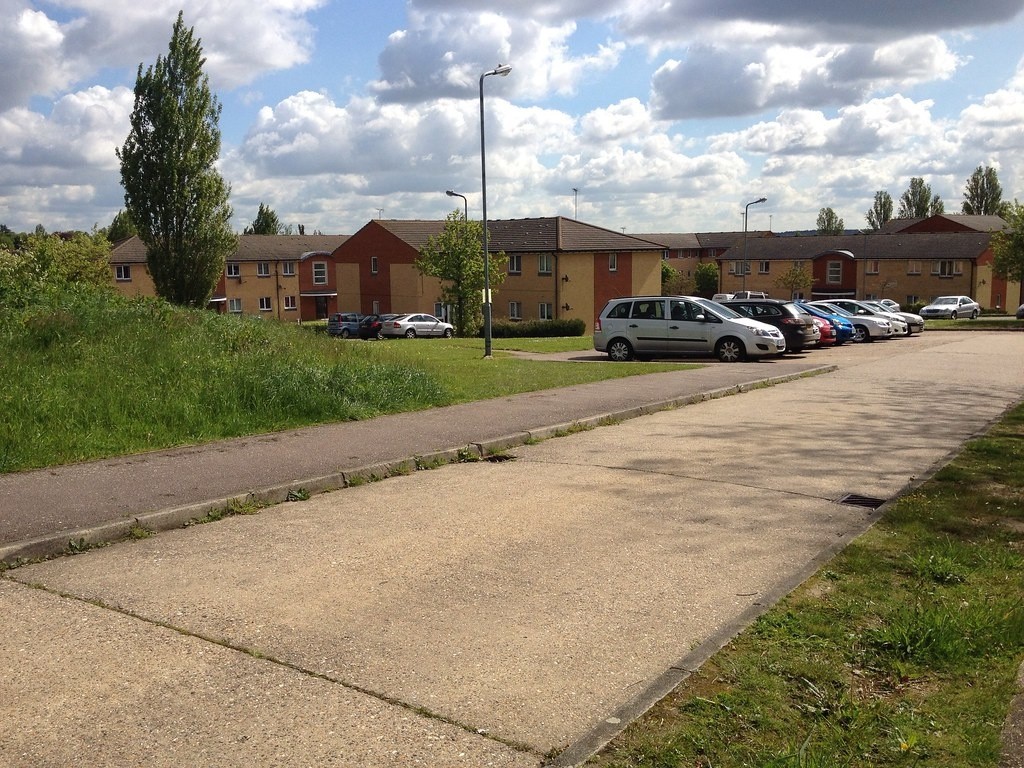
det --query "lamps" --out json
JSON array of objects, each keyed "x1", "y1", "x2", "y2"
[
  {"x1": 562, "y1": 303, "x2": 569, "y2": 311},
  {"x1": 562, "y1": 275, "x2": 569, "y2": 282}
]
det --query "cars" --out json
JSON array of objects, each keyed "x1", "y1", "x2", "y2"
[
  {"x1": 919, "y1": 295, "x2": 981, "y2": 320},
  {"x1": 380, "y1": 313, "x2": 455, "y2": 340},
  {"x1": 1016, "y1": 304, "x2": 1024, "y2": 319},
  {"x1": 710, "y1": 292, "x2": 925, "y2": 353}
]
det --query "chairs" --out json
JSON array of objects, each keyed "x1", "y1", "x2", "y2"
[
  {"x1": 671, "y1": 306, "x2": 685, "y2": 320},
  {"x1": 647, "y1": 305, "x2": 655, "y2": 318}
]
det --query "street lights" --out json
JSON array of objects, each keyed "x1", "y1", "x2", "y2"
[
  {"x1": 446, "y1": 191, "x2": 468, "y2": 244},
  {"x1": 743, "y1": 198, "x2": 768, "y2": 291},
  {"x1": 572, "y1": 188, "x2": 577, "y2": 223},
  {"x1": 478, "y1": 63, "x2": 511, "y2": 355}
]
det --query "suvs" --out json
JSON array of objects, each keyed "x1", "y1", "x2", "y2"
[
  {"x1": 357, "y1": 313, "x2": 400, "y2": 339},
  {"x1": 592, "y1": 295, "x2": 787, "y2": 365}
]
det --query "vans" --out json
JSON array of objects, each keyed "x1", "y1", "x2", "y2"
[{"x1": 328, "y1": 312, "x2": 367, "y2": 339}]
